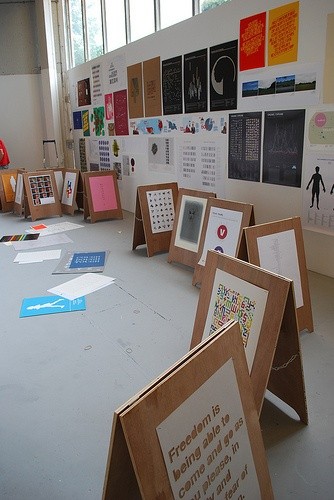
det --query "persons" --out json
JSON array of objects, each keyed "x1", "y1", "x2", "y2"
[{"x1": 0, "y1": 140, "x2": 10, "y2": 170}]
